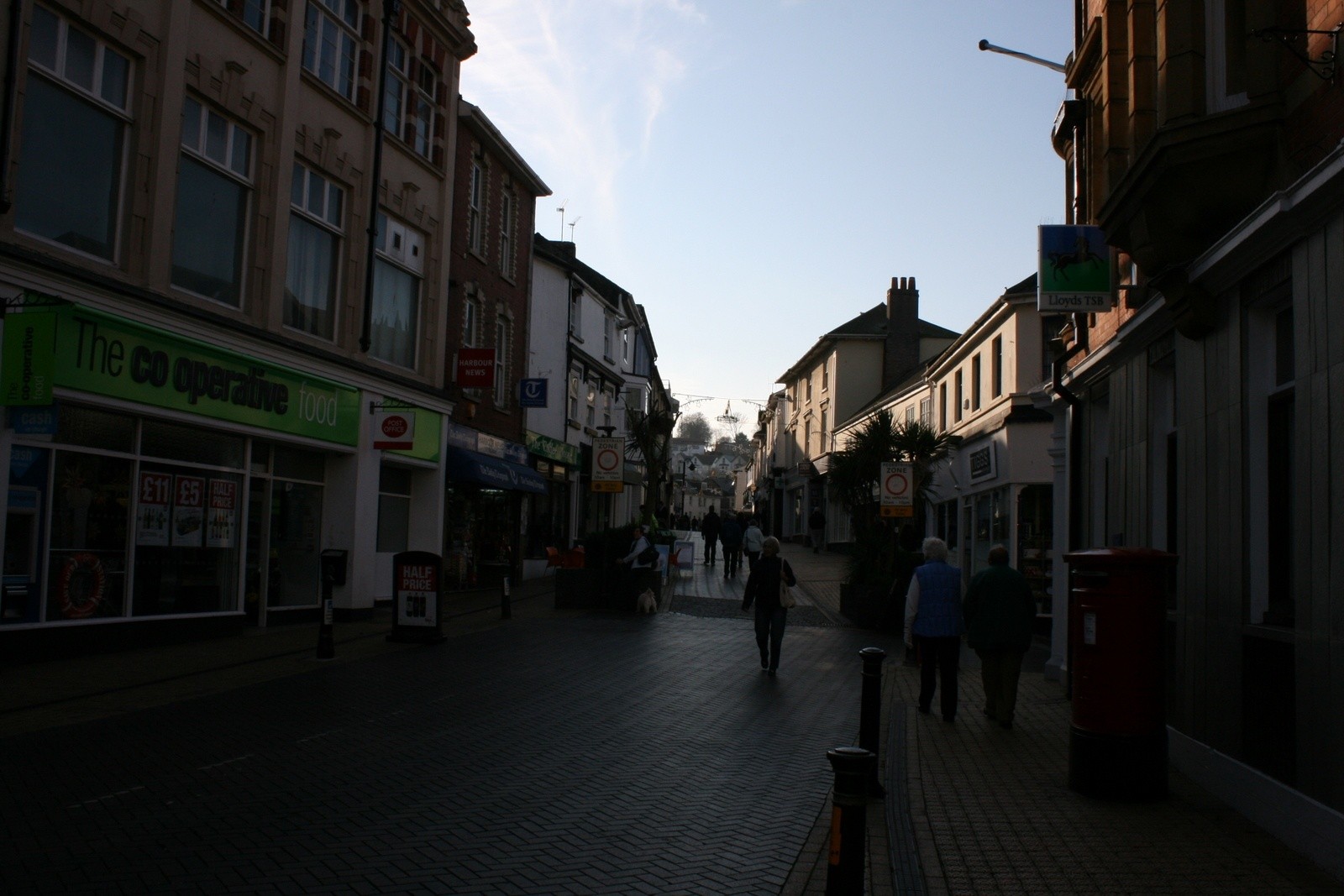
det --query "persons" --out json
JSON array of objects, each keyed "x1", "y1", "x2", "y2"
[
  {"x1": 741, "y1": 536, "x2": 798, "y2": 674},
  {"x1": 903, "y1": 537, "x2": 968, "y2": 723},
  {"x1": 611, "y1": 506, "x2": 771, "y2": 610},
  {"x1": 964, "y1": 544, "x2": 1037, "y2": 727}
]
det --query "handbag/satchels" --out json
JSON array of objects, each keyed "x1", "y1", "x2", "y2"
[{"x1": 778, "y1": 559, "x2": 797, "y2": 611}]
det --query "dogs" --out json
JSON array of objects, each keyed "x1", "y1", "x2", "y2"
[{"x1": 637, "y1": 588, "x2": 658, "y2": 614}]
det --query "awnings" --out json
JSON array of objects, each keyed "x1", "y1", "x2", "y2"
[{"x1": 446, "y1": 448, "x2": 548, "y2": 494}]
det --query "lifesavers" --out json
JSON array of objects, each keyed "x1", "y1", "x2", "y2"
[{"x1": 58, "y1": 553, "x2": 106, "y2": 619}]
